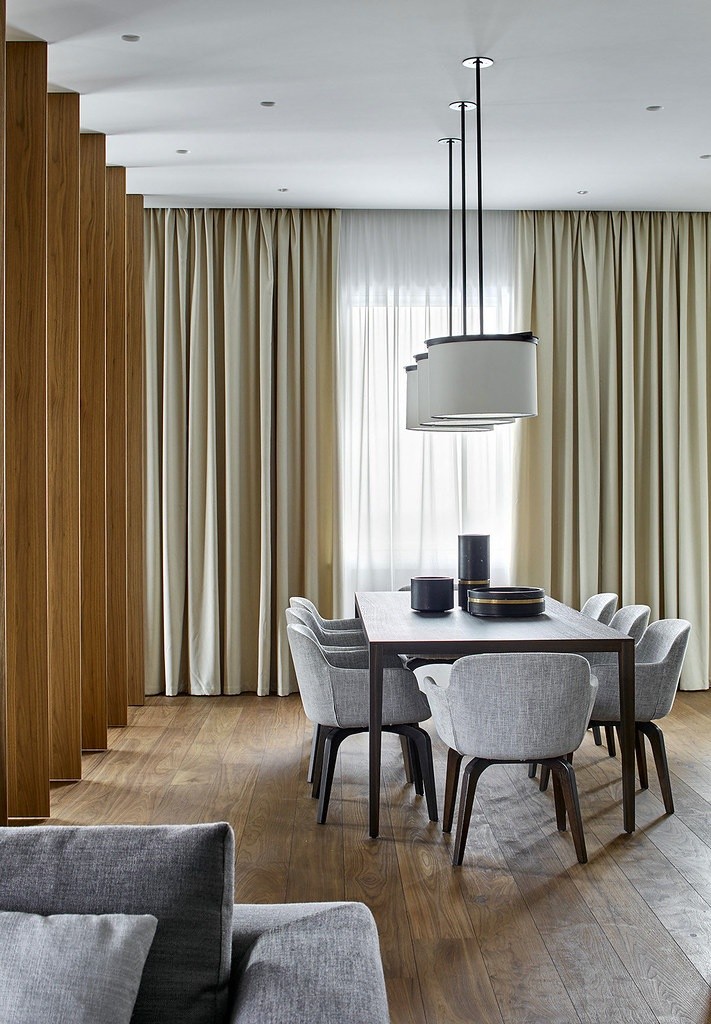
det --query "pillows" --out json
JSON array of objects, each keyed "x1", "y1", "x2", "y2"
[
  {"x1": 0, "y1": 911, "x2": 160, "y2": 1024},
  {"x1": 0, "y1": 820, "x2": 237, "y2": 1024}
]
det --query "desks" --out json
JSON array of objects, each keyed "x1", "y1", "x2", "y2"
[{"x1": 352, "y1": 590, "x2": 638, "y2": 836}]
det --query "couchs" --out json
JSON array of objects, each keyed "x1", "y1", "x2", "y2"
[{"x1": 230, "y1": 900, "x2": 392, "y2": 1024}]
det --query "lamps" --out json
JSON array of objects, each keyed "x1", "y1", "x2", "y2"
[
  {"x1": 413, "y1": 99, "x2": 517, "y2": 427},
  {"x1": 403, "y1": 136, "x2": 495, "y2": 433},
  {"x1": 424, "y1": 58, "x2": 540, "y2": 419}
]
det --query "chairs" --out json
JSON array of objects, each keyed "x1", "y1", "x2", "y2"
[
  {"x1": 527, "y1": 593, "x2": 694, "y2": 814},
  {"x1": 284, "y1": 596, "x2": 439, "y2": 825},
  {"x1": 421, "y1": 651, "x2": 600, "y2": 868}
]
